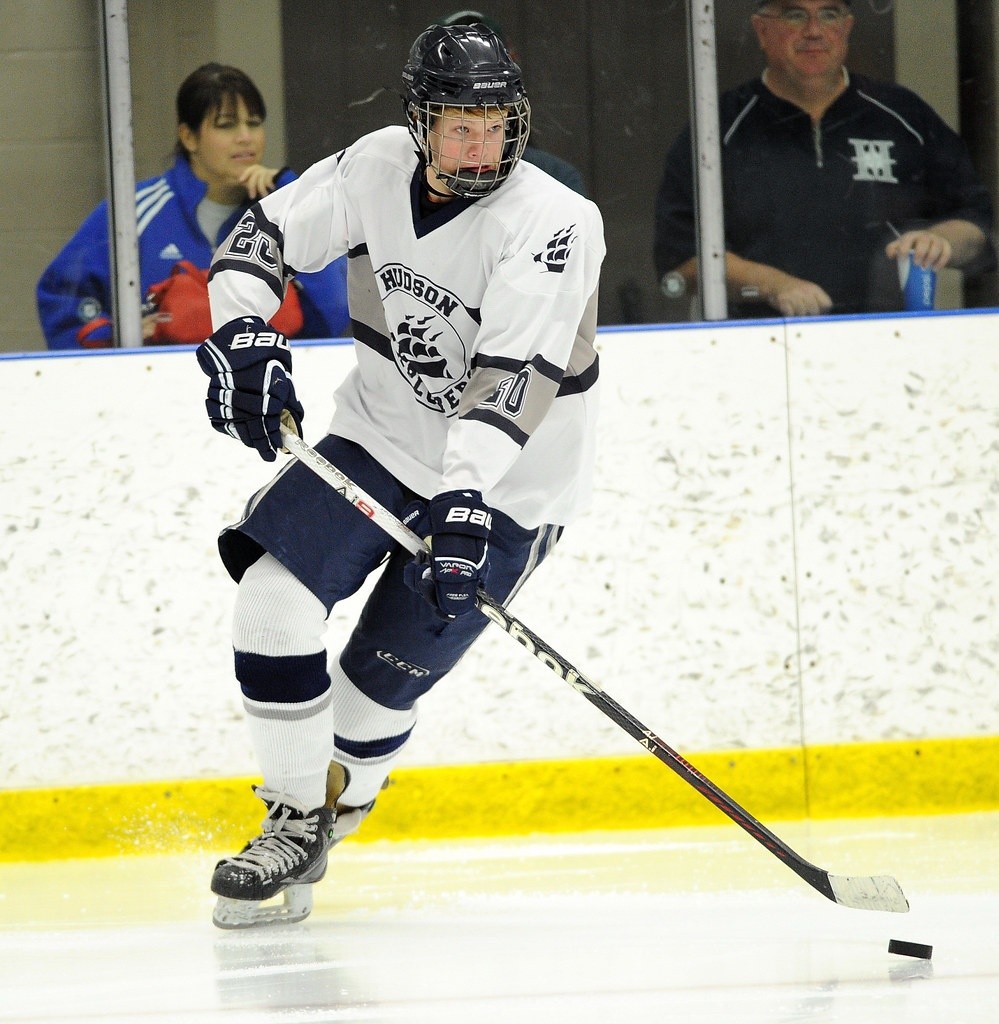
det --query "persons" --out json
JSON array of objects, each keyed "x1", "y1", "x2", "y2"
[
  {"x1": 37, "y1": 62, "x2": 349, "y2": 349},
  {"x1": 653, "y1": 0, "x2": 993, "y2": 317},
  {"x1": 195, "y1": 12, "x2": 608, "y2": 930}
]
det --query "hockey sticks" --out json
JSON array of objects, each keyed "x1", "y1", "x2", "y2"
[{"x1": 277, "y1": 417, "x2": 909, "y2": 915}]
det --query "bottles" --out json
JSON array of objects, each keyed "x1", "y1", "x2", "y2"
[{"x1": 739, "y1": 287, "x2": 765, "y2": 319}]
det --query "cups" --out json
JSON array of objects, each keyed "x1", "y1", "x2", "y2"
[{"x1": 898, "y1": 248, "x2": 937, "y2": 312}]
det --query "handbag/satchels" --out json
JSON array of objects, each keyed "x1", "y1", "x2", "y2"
[{"x1": 150, "y1": 258, "x2": 305, "y2": 345}]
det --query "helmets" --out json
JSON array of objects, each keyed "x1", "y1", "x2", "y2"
[{"x1": 400, "y1": 11, "x2": 531, "y2": 202}]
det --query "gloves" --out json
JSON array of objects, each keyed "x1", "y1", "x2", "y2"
[
  {"x1": 400, "y1": 492, "x2": 492, "y2": 630},
  {"x1": 196, "y1": 317, "x2": 305, "y2": 464}
]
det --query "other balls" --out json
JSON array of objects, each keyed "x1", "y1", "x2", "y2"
[{"x1": 888, "y1": 938, "x2": 933, "y2": 960}]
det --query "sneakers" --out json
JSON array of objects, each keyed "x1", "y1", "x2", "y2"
[{"x1": 207, "y1": 762, "x2": 388, "y2": 929}]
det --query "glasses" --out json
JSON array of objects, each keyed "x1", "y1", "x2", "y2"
[{"x1": 755, "y1": 7, "x2": 852, "y2": 25}]
